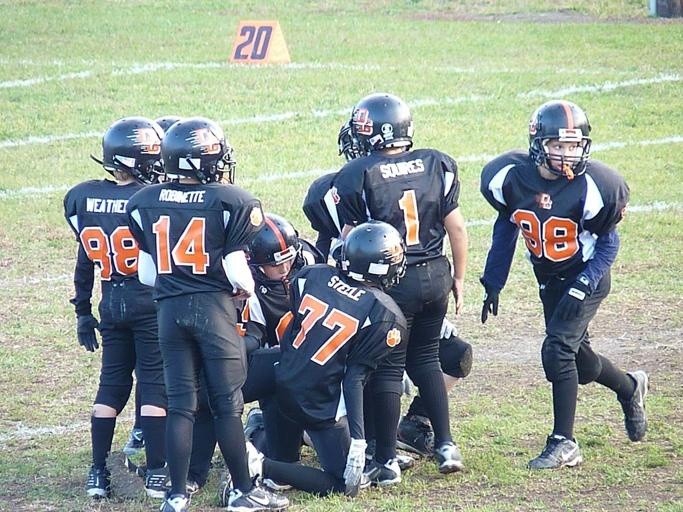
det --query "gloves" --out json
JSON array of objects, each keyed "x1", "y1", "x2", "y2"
[
  {"x1": 343, "y1": 438, "x2": 367, "y2": 487},
  {"x1": 481, "y1": 288, "x2": 499, "y2": 323},
  {"x1": 440, "y1": 317, "x2": 457, "y2": 340},
  {"x1": 558, "y1": 280, "x2": 591, "y2": 321},
  {"x1": 77, "y1": 315, "x2": 99, "y2": 352}
]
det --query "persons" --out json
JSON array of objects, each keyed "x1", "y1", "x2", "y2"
[
  {"x1": 222, "y1": 213, "x2": 406, "y2": 511},
  {"x1": 61, "y1": 116, "x2": 200, "y2": 503},
  {"x1": 478, "y1": 97, "x2": 650, "y2": 471},
  {"x1": 124, "y1": 115, "x2": 293, "y2": 512},
  {"x1": 302, "y1": 92, "x2": 472, "y2": 507},
  {"x1": 118, "y1": 114, "x2": 216, "y2": 497}
]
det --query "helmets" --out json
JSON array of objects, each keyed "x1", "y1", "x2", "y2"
[
  {"x1": 336, "y1": 219, "x2": 408, "y2": 291},
  {"x1": 101, "y1": 114, "x2": 236, "y2": 185},
  {"x1": 338, "y1": 93, "x2": 415, "y2": 163},
  {"x1": 528, "y1": 100, "x2": 592, "y2": 177},
  {"x1": 244, "y1": 212, "x2": 303, "y2": 265}
]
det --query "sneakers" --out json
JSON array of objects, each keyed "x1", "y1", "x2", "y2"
[
  {"x1": 122, "y1": 429, "x2": 146, "y2": 454},
  {"x1": 435, "y1": 441, "x2": 463, "y2": 473},
  {"x1": 144, "y1": 467, "x2": 198, "y2": 512},
  {"x1": 528, "y1": 434, "x2": 580, "y2": 468},
  {"x1": 365, "y1": 415, "x2": 436, "y2": 484},
  {"x1": 86, "y1": 468, "x2": 111, "y2": 498},
  {"x1": 618, "y1": 370, "x2": 650, "y2": 442},
  {"x1": 220, "y1": 407, "x2": 289, "y2": 512}
]
